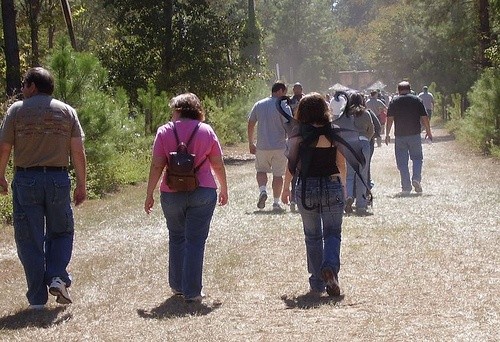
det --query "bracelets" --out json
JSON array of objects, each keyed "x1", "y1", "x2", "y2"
[
  {"x1": 385, "y1": 136, "x2": 390, "y2": 139},
  {"x1": 249, "y1": 143, "x2": 254, "y2": 147}
]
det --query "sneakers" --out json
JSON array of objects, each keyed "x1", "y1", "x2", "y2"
[
  {"x1": 29, "y1": 305, "x2": 46, "y2": 310},
  {"x1": 49, "y1": 277, "x2": 73, "y2": 304},
  {"x1": 257, "y1": 190, "x2": 267, "y2": 208}
]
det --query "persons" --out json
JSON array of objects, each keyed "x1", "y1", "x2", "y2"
[
  {"x1": 290, "y1": 82, "x2": 306, "y2": 114},
  {"x1": 384, "y1": 81, "x2": 432, "y2": 195},
  {"x1": 0, "y1": 67, "x2": 87, "y2": 309},
  {"x1": 247, "y1": 83, "x2": 294, "y2": 212},
  {"x1": 419, "y1": 85, "x2": 435, "y2": 139},
  {"x1": 144, "y1": 92, "x2": 229, "y2": 301},
  {"x1": 326, "y1": 89, "x2": 388, "y2": 215},
  {"x1": 275, "y1": 91, "x2": 373, "y2": 298}
]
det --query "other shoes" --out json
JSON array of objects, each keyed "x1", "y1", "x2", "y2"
[
  {"x1": 345, "y1": 196, "x2": 353, "y2": 213},
  {"x1": 273, "y1": 203, "x2": 286, "y2": 211},
  {"x1": 412, "y1": 179, "x2": 423, "y2": 193},
  {"x1": 185, "y1": 293, "x2": 205, "y2": 302},
  {"x1": 171, "y1": 288, "x2": 183, "y2": 296},
  {"x1": 321, "y1": 267, "x2": 340, "y2": 297}
]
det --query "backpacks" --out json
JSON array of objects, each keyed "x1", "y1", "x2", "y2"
[{"x1": 165, "y1": 121, "x2": 208, "y2": 192}]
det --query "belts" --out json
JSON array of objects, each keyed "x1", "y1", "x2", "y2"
[{"x1": 16, "y1": 166, "x2": 68, "y2": 172}]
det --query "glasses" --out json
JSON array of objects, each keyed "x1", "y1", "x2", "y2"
[{"x1": 21, "y1": 81, "x2": 25, "y2": 89}]
